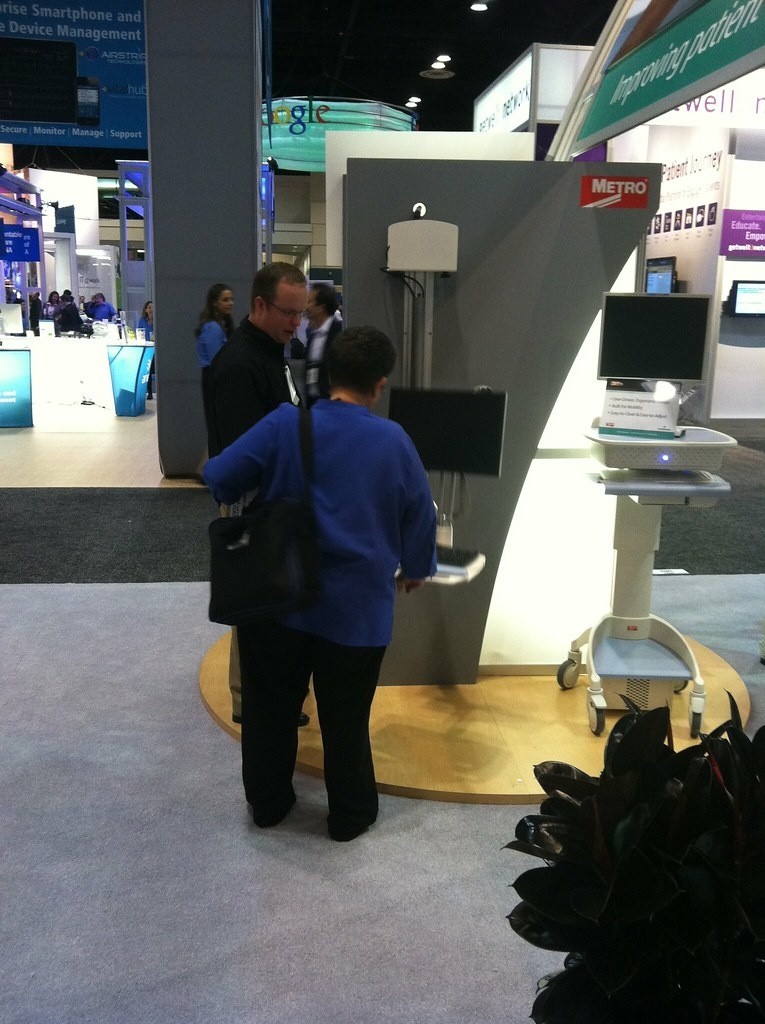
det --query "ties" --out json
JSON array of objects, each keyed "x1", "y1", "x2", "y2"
[{"x1": 285, "y1": 364, "x2": 300, "y2": 406}]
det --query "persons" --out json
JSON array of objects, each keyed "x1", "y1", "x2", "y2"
[
  {"x1": 138, "y1": 301, "x2": 155, "y2": 399},
  {"x1": 195, "y1": 284, "x2": 234, "y2": 368},
  {"x1": 205, "y1": 325, "x2": 437, "y2": 840},
  {"x1": 205, "y1": 261, "x2": 307, "y2": 459},
  {"x1": 55, "y1": 294, "x2": 82, "y2": 331},
  {"x1": 80, "y1": 293, "x2": 117, "y2": 321},
  {"x1": 284, "y1": 283, "x2": 343, "y2": 410},
  {"x1": 6, "y1": 287, "x2": 71, "y2": 329}
]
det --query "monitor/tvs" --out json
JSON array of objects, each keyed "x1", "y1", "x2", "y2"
[
  {"x1": 597, "y1": 292, "x2": 713, "y2": 382},
  {"x1": 730, "y1": 280, "x2": 765, "y2": 318},
  {"x1": 387, "y1": 386, "x2": 509, "y2": 480},
  {"x1": 645, "y1": 256, "x2": 677, "y2": 293}
]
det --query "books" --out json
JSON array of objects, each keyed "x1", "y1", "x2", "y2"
[{"x1": 436, "y1": 544, "x2": 480, "y2": 573}]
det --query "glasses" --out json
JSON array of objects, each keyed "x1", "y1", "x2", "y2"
[{"x1": 263, "y1": 299, "x2": 309, "y2": 319}]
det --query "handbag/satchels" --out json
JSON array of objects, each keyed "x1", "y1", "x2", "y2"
[{"x1": 208, "y1": 489, "x2": 322, "y2": 626}]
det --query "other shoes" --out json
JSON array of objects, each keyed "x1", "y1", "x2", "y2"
[
  {"x1": 147, "y1": 394, "x2": 153, "y2": 399},
  {"x1": 232, "y1": 711, "x2": 309, "y2": 726}
]
